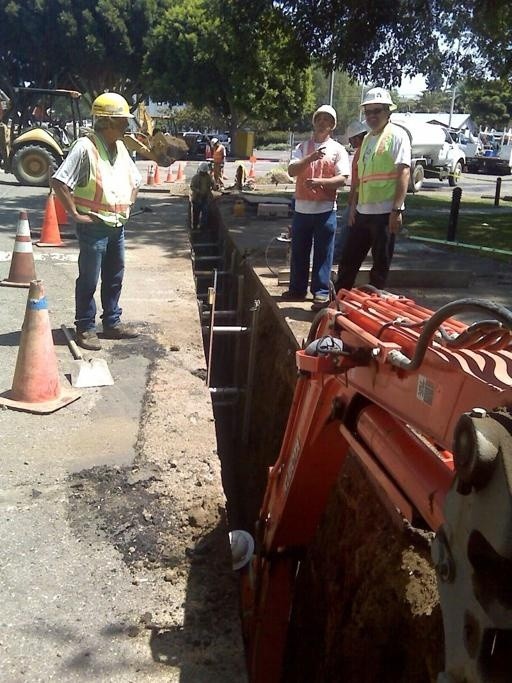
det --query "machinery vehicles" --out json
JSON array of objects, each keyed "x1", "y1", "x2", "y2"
[
  {"x1": 347, "y1": 120, "x2": 466, "y2": 192},
  {"x1": 466, "y1": 125, "x2": 511, "y2": 176},
  {"x1": 1, "y1": 86, "x2": 189, "y2": 187},
  {"x1": 239, "y1": 286, "x2": 510, "y2": 680}
]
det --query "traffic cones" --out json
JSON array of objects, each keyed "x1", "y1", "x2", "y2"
[
  {"x1": 164, "y1": 165, "x2": 175, "y2": 183},
  {"x1": 144, "y1": 165, "x2": 160, "y2": 186},
  {"x1": 54, "y1": 194, "x2": 71, "y2": 225},
  {"x1": 34, "y1": 189, "x2": 67, "y2": 246},
  {"x1": 0, "y1": 280, "x2": 84, "y2": 415},
  {"x1": 247, "y1": 161, "x2": 256, "y2": 178},
  {"x1": 0, "y1": 211, "x2": 41, "y2": 287},
  {"x1": 174, "y1": 164, "x2": 185, "y2": 184}
]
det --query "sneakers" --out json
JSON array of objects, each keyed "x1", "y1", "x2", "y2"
[
  {"x1": 313, "y1": 294, "x2": 330, "y2": 303},
  {"x1": 104, "y1": 323, "x2": 140, "y2": 338},
  {"x1": 282, "y1": 291, "x2": 308, "y2": 300},
  {"x1": 75, "y1": 327, "x2": 101, "y2": 350}
]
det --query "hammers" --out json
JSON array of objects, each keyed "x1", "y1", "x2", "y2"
[{"x1": 130, "y1": 206, "x2": 155, "y2": 216}]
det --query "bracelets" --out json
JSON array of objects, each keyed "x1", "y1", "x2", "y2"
[{"x1": 391, "y1": 207, "x2": 405, "y2": 214}]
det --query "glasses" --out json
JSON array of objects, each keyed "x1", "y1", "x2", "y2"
[{"x1": 364, "y1": 107, "x2": 386, "y2": 116}]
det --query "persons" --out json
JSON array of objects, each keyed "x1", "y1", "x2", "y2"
[
  {"x1": 333, "y1": 87, "x2": 412, "y2": 295},
  {"x1": 52, "y1": 92, "x2": 139, "y2": 349},
  {"x1": 33, "y1": 95, "x2": 69, "y2": 148},
  {"x1": 281, "y1": 103, "x2": 351, "y2": 303},
  {"x1": 226, "y1": 529, "x2": 256, "y2": 630},
  {"x1": 210, "y1": 137, "x2": 227, "y2": 191},
  {"x1": 191, "y1": 162, "x2": 219, "y2": 229}
]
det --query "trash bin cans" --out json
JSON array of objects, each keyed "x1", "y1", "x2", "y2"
[{"x1": 233, "y1": 130, "x2": 255, "y2": 157}]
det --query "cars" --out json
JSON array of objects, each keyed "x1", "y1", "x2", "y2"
[{"x1": 178, "y1": 132, "x2": 228, "y2": 159}]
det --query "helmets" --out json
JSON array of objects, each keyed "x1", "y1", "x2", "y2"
[
  {"x1": 313, "y1": 104, "x2": 338, "y2": 130},
  {"x1": 211, "y1": 137, "x2": 218, "y2": 145},
  {"x1": 347, "y1": 121, "x2": 368, "y2": 141},
  {"x1": 361, "y1": 87, "x2": 397, "y2": 111},
  {"x1": 229, "y1": 529, "x2": 256, "y2": 571},
  {"x1": 199, "y1": 162, "x2": 209, "y2": 172},
  {"x1": 92, "y1": 93, "x2": 136, "y2": 118}
]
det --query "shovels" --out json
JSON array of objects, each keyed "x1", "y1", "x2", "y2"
[{"x1": 60, "y1": 324, "x2": 115, "y2": 388}]
